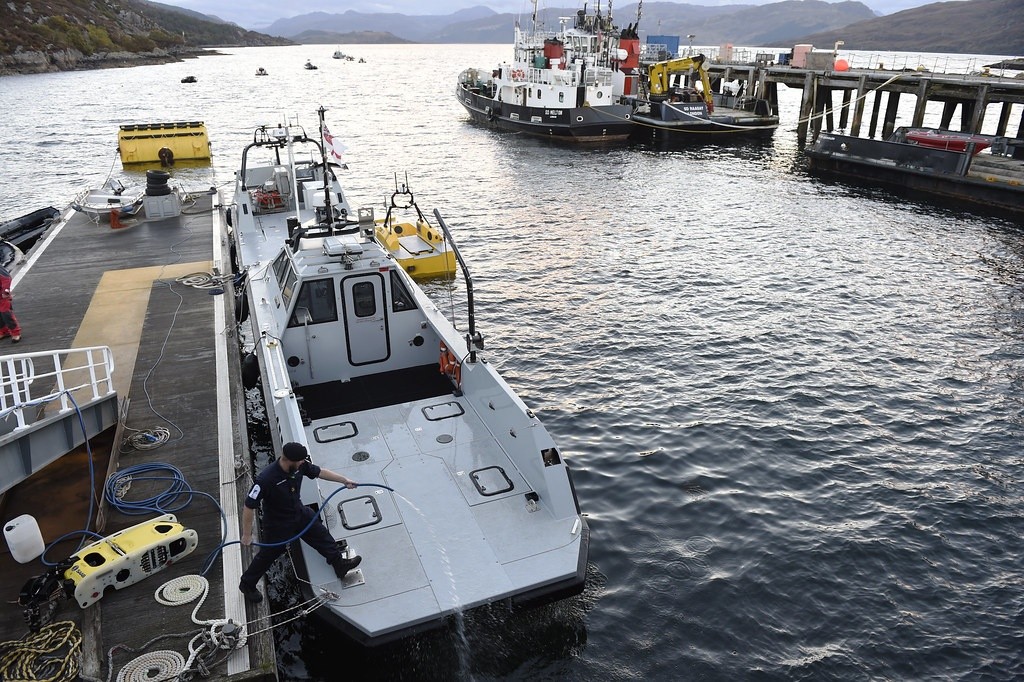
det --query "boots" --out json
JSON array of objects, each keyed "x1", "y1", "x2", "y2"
[
  {"x1": 332, "y1": 556, "x2": 362, "y2": 580},
  {"x1": 239, "y1": 577, "x2": 262, "y2": 603}
]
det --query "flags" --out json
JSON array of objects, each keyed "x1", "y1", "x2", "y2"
[
  {"x1": 322, "y1": 121, "x2": 348, "y2": 168},
  {"x1": 597, "y1": 26, "x2": 601, "y2": 44}
]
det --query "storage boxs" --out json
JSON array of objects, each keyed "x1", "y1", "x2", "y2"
[
  {"x1": 779, "y1": 53, "x2": 793, "y2": 64},
  {"x1": 302, "y1": 181, "x2": 325, "y2": 209}
]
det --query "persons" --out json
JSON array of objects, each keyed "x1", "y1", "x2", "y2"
[
  {"x1": 0, "y1": 264, "x2": 21, "y2": 343},
  {"x1": 239, "y1": 443, "x2": 362, "y2": 604}
]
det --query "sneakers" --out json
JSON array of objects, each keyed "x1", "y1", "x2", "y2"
[
  {"x1": 12, "y1": 334, "x2": 21, "y2": 342},
  {"x1": 0, "y1": 330, "x2": 11, "y2": 339}
]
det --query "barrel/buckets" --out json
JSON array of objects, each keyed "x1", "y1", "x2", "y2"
[{"x1": 3, "y1": 514, "x2": 45, "y2": 564}]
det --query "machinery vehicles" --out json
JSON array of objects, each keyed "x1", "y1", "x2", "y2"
[{"x1": 650, "y1": 54, "x2": 716, "y2": 114}]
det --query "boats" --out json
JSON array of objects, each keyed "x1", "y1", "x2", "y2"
[
  {"x1": 803, "y1": 126, "x2": 1024, "y2": 225},
  {"x1": 225, "y1": 106, "x2": 589, "y2": 650},
  {"x1": 72, "y1": 178, "x2": 145, "y2": 222},
  {"x1": 0, "y1": 206, "x2": 60, "y2": 279},
  {"x1": 332, "y1": 46, "x2": 346, "y2": 59},
  {"x1": 374, "y1": 171, "x2": 456, "y2": 279},
  {"x1": 346, "y1": 56, "x2": 355, "y2": 61},
  {"x1": 905, "y1": 131, "x2": 991, "y2": 154},
  {"x1": 456, "y1": 0, "x2": 635, "y2": 148},
  {"x1": 181, "y1": 76, "x2": 197, "y2": 83},
  {"x1": 255, "y1": 68, "x2": 268, "y2": 76},
  {"x1": 359, "y1": 59, "x2": 366, "y2": 63},
  {"x1": 304, "y1": 59, "x2": 317, "y2": 69}
]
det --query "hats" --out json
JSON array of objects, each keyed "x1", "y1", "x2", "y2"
[{"x1": 283, "y1": 442, "x2": 307, "y2": 462}]
